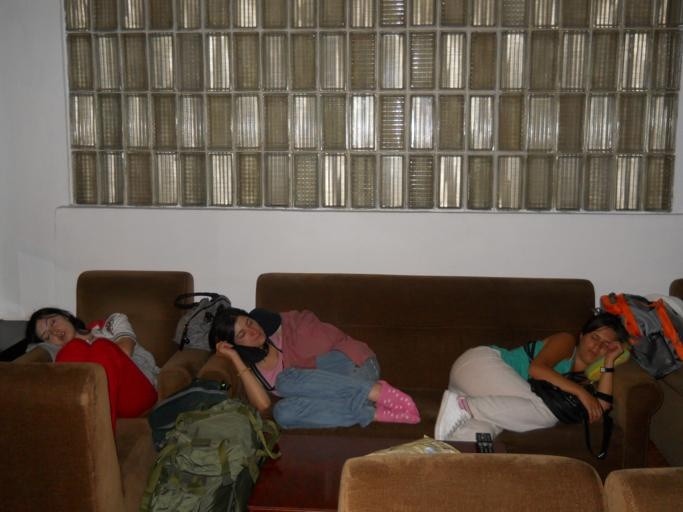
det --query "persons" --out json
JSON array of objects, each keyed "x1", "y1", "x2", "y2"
[
  {"x1": 210, "y1": 304, "x2": 423, "y2": 428},
  {"x1": 14, "y1": 305, "x2": 160, "y2": 438},
  {"x1": 433, "y1": 312, "x2": 627, "y2": 443}
]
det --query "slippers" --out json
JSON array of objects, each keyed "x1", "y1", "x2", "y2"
[
  {"x1": 375, "y1": 379, "x2": 420, "y2": 416},
  {"x1": 374, "y1": 403, "x2": 420, "y2": 425}
]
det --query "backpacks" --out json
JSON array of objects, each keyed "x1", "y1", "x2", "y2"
[
  {"x1": 599, "y1": 293, "x2": 683, "y2": 380},
  {"x1": 139, "y1": 463, "x2": 251, "y2": 512},
  {"x1": 153, "y1": 397, "x2": 280, "y2": 486}
]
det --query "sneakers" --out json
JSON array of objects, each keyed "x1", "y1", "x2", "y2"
[{"x1": 433, "y1": 389, "x2": 471, "y2": 440}]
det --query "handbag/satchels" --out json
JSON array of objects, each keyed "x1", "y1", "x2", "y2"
[
  {"x1": 173, "y1": 292, "x2": 230, "y2": 352},
  {"x1": 526, "y1": 372, "x2": 595, "y2": 424}
]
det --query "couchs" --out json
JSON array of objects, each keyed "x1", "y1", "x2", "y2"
[{"x1": 209, "y1": 271, "x2": 664, "y2": 469}]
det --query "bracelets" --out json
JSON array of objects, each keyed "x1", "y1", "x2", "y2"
[
  {"x1": 237, "y1": 365, "x2": 253, "y2": 375},
  {"x1": 599, "y1": 365, "x2": 614, "y2": 374}
]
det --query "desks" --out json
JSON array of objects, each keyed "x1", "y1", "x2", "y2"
[{"x1": 244, "y1": 429, "x2": 507, "y2": 510}]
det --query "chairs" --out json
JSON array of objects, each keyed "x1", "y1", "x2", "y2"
[{"x1": 1, "y1": 269, "x2": 212, "y2": 511}]
